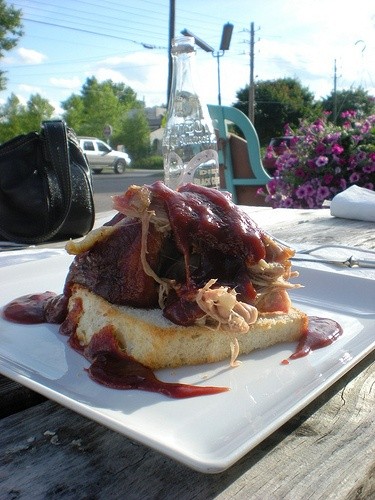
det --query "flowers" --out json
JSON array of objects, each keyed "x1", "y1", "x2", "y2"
[{"x1": 256, "y1": 110, "x2": 375, "y2": 209}]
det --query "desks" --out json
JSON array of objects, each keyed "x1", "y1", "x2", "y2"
[{"x1": 0, "y1": 205, "x2": 375, "y2": 500}]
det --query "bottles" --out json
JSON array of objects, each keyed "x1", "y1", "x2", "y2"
[{"x1": 162, "y1": 36, "x2": 220, "y2": 192}]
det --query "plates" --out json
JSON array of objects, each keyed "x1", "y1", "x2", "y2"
[{"x1": 0, "y1": 249, "x2": 375, "y2": 474}]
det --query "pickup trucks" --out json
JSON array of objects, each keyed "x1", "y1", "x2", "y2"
[{"x1": 77, "y1": 137, "x2": 130, "y2": 175}]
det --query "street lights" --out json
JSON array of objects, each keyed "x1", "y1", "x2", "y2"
[{"x1": 182, "y1": 22, "x2": 235, "y2": 106}]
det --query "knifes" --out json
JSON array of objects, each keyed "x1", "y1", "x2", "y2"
[{"x1": 289, "y1": 254, "x2": 375, "y2": 268}]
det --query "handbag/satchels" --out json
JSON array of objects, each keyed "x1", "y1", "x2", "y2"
[{"x1": 0, "y1": 119, "x2": 95, "y2": 244}]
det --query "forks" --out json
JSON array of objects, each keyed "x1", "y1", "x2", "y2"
[{"x1": 264, "y1": 231, "x2": 375, "y2": 253}]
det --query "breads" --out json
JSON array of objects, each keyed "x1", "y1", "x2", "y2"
[{"x1": 66, "y1": 285, "x2": 309, "y2": 369}]
